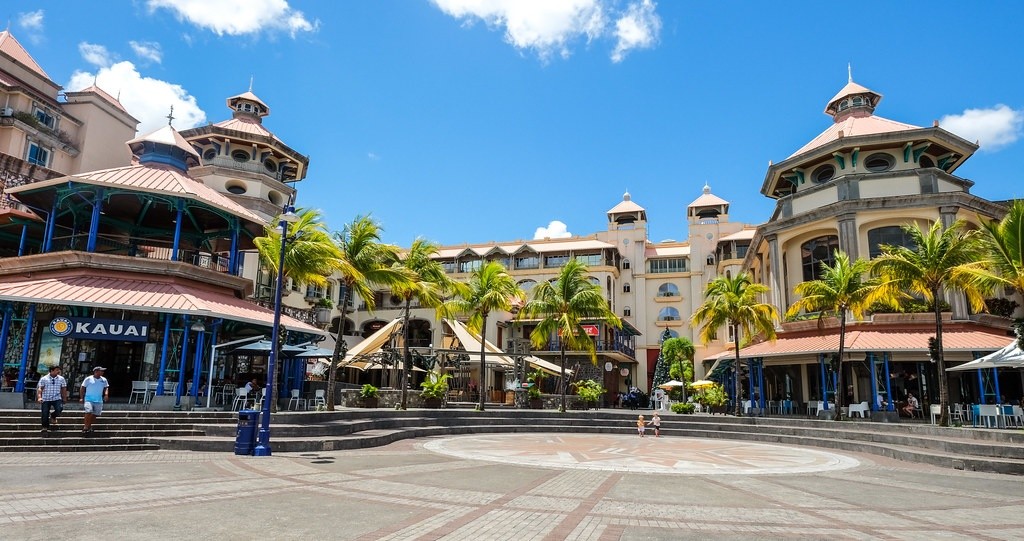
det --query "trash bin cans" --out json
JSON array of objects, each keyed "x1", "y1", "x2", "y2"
[{"x1": 234, "y1": 410, "x2": 261, "y2": 454}]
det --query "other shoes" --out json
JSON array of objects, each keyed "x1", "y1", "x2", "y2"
[
  {"x1": 49, "y1": 418, "x2": 58, "y2": 423},
  {"x1": 910, "y1": 416, "x2": 916, "y2": 419},
  {"x1": 638, "y1": 434, "x2": 644, "y2": 437},
  {"x1": 42, "y1": 427, "x2": 47, "y2": 434},
  {"x1": 656, "y1": 435, "x2": 660, "y2": 437},
  {"x1": 83, "y1": 427, "x2": 93, "y2": 434}
]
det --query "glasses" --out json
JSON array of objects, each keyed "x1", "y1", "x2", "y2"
[
  {"x1": 908, "y1": 395, "x2": 912, "y2": 396},
  {"x1": 52, "y1": 378, "x2": 55, "y2": 385}
]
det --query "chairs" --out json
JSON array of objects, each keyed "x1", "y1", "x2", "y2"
[
  {"x1": 308, "y1": 390, "x2": 326, "y2": 409},
  {"x1": 148, "y1": 381, "x2": 159, "y2": 404},
  {"x1": 186, "y1": 383, "x2": 204, "y2": 396},
  {"x1": 234, "y1": 388, "x2": 256, "y2": 410},
  {"x1": 906, "y1": 395, "x2": 1024, "y2": 432},
  {"x1": 288, "y1": 389, "x2": 307, "y2": 411},
  {"x1": 231, "y1": 389, "x2": 241, "y2": 410},
  {"x1": 214, "y1": 384, "x2": 236, "y2": 405},
  {"x1": 729, "y1": 395, "x2": 871, "y2": 418},
  {"x1": 161, "y1": 381, "x2": 179, "y2": 396},
  {"x1": 260, "y1": 388, "x2": 267, "y2": 412},
  {"x1": 128, "y1": 381, "x2": 150, "y2": 405}
]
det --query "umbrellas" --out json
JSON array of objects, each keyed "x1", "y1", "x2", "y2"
[
  {"x1": 690, "y1": 379, "x2": 718, "y2": 399},
  {"x1": 215, "y1": 334, "x2": 427, "y2": 397},
  {"x1": 657, "y1": 380, "x2": 687, "y2": 389},
  {"x1": 944, "y1": 336, "x2": 1024, "y2": 372}
]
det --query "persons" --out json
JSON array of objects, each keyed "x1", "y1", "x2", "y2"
[
  {"x1": 6, "y1": 367, "x2": 18, "y2": 390},
  {"x1": 244, "y1": 378, "x2": 260, "y2": 410},
  {"x1": 1001, "y1": 395, "x2": 1009, "y2": 404},
  {"x1": 647, "y1": 413, "x2": 661, "y2": 437},
  {"x1": 37, "y1": 365, "x2": 68, "y2": 432},
  {"x1": 25, "y1": 365, "x2": 40, "y2": 401},
  {"x1": 613, "y1": 391, "x2": 655, "y2": 410},
  {"x1": 902, "y1": 393, "x2": 919, "y2": 420},
  {"x1": 79, "y1": 366, "x2": 110, "y2": 433},
  {"x1": 637, "y1": 415, "x2": 647, "y2": 438}
]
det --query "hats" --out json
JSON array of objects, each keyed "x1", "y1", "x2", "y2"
[{"x1": 93, "y1": 366, "x2": 107, "y2": 372}]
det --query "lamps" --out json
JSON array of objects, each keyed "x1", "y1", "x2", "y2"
[{"x1": 190, "y1": 316, "x2": 205, "y2": 331}]
